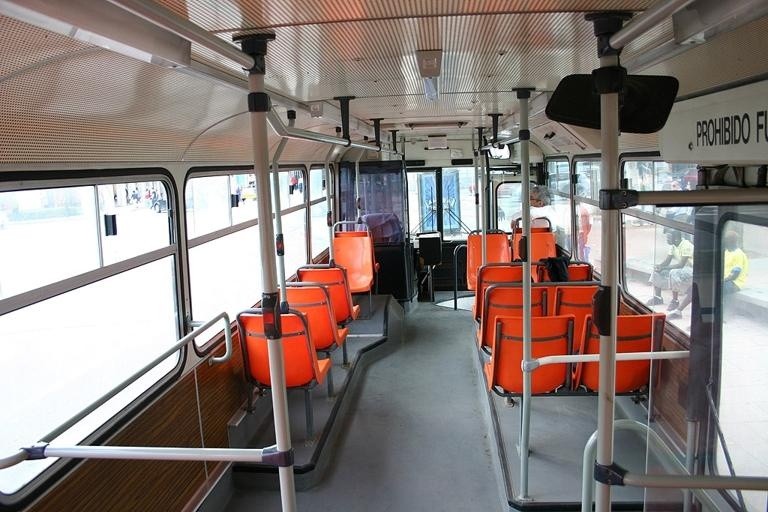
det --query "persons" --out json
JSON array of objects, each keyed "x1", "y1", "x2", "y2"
[
  {"x1": 507, "y1": 183, "x2": 558, "y2": 234},
  {"x1": 289, "y1": 174, "x2": 297, "y2": 195},
  {"x1": 666, "y1": 230, "x2": 749, "y2": 331},
  {"x1": 646, "y1": 228, "x2": 694, "y2": 310},
  {"x1": 569, "y1": 199, "x2": 592, "y2": 261}
]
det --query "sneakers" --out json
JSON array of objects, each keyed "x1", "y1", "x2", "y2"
[
  {"x1": 667, "y1": 299, "x2": 683, "y2": 319},
  {"x1": 645, "y1": 295, "x2": 664, "y2": 306}
]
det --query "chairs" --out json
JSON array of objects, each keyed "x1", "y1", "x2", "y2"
[
  {"x1": 466, "y1": 216, "x2": 664, "y2": 454},
  {"x1": 237, "y1": 308, "x2": 333, "y2": 448},
  {"x1": 276, "y1": 282, "x2": 348, "y2": 396},
  {"x1": 330, "y1": 221, "x2": 380, "y2": 320},
  {"x1": 296, "y1": 263, "x2": 361, "y2": 366}
]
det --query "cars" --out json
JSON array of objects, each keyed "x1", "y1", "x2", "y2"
[
  {"x1": 469, "y1": 182, "x2": 512, "y2": 197},
  {"x1": 154, "y1": 194, "x2": 167, "y2": 213},
  {"x1": 549, "y1": 162, "x2": 599, "y2": 196},
  {"x1": 240, "y1": 188, "x2": 257, "y2": 200},
  {"x1": 643, "y1": 162, "x2": 698, "y2": 190}
]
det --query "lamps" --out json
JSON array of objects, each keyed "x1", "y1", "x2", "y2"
[{"x1": 415, "y1": 49, "x2": 442, "y2": 101}]
